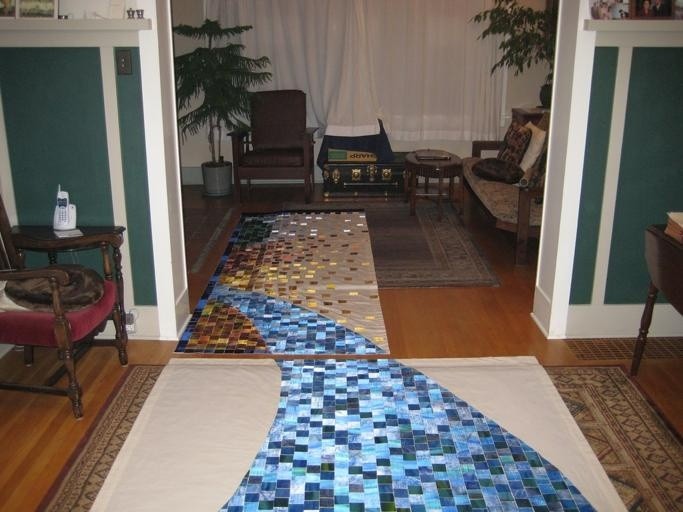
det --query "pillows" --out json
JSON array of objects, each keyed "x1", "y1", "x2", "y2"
[
  {"x1": 494, "y1": 118, "x2": 532, "y2": 167},
  {"x1": 511, "y1": 120, "x2": 546, "y2": 188},
  {"x1": 470, "y1": 157, "x2": 524, "y2": 185}
]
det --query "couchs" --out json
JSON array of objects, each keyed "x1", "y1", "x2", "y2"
[{"x1": 459, "y1": 112, "x2": 551, "y2": 265}]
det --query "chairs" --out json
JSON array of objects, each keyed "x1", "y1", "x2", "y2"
[
  {"x1": 627, "y1": 222, "x2": 682, "y2": 379},
  {"x1": 0, "y1": 191, "x2": 128, "y2": 420},
  {"x1": 226, "y1": 89, "x2": 321, "y2": 206}
]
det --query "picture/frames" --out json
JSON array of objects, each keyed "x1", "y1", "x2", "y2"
[
  {"x1": 625, "y1": 0, "x2": 675, "y2": 20},
  {"x1": 14, "y1": 0, "x2": 59, "y2": 20}
]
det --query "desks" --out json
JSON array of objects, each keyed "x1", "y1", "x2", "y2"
[
  {"x1": 402, "y1": 148, "x2": 464, "y2": 222},
  {"x1": 509, "y1": 107, "x2": 550, "y2": 125}
]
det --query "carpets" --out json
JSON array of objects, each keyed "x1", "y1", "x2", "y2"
[
  {"x1": 30, "y1": 363, "x2": 682, "y2": 512},
  {"x1": 279, "y1": 197, "x2": 502, "y2": 291},
  {"x1": 86, "y1": 354, "x2": 629, "y2": 512},
  {"x1": 171, "y1": 206, "x2": 391, "y2": 357}
]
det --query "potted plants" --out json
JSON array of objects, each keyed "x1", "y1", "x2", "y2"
[
  {"x1": 169, "y1": 15, "x2": 273, "y2": 198},
  {"x1": 465, "y1": 0, "x2": 559, "y2": 107}
]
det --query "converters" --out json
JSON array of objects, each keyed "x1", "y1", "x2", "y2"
[{"x1": 125, "y1": 313, "x2": 133, "y2": 325}]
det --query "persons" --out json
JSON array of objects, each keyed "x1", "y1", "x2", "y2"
[{"x1": 588, "y1": 0, "x2": 674, "y2": 21}]
